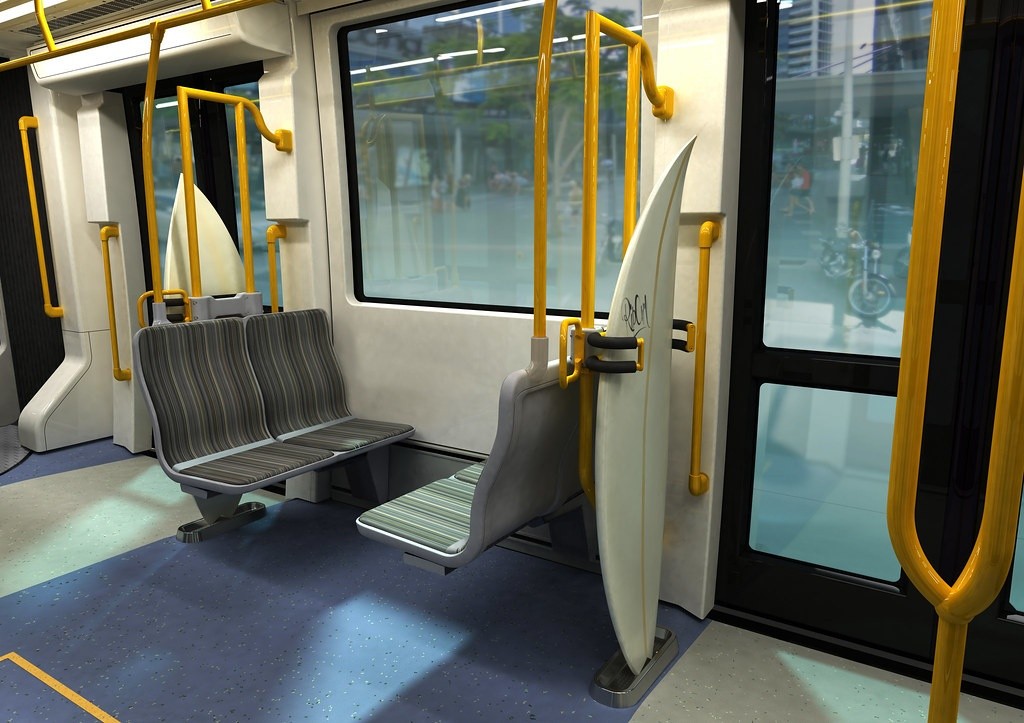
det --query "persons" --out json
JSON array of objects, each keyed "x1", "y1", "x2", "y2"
[
  {"x1": 430, "y1": 165, "x2": 614, "y2": 220},
  {"x1": 858, "y1": 144, "x2": 910, "y2": 175},
  {"x1": 780, "y1": 154, "x2": 816, "y2": 217}
]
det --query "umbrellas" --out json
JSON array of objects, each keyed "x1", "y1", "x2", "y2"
[{"x1": 598, "y1": 158, "x2": 612, "y2": 165}]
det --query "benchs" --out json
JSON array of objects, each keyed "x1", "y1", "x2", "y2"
[
  {"x1": 132, "y1": 309, "x2": 415, "y2": 544},
  {"x1": 356, "y1": 358, "x2": 599, "y2": 575}
]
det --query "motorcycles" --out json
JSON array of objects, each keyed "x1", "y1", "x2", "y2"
[{"x1": 819, "y1": 228, "x2": 897, "y2": 317}]
